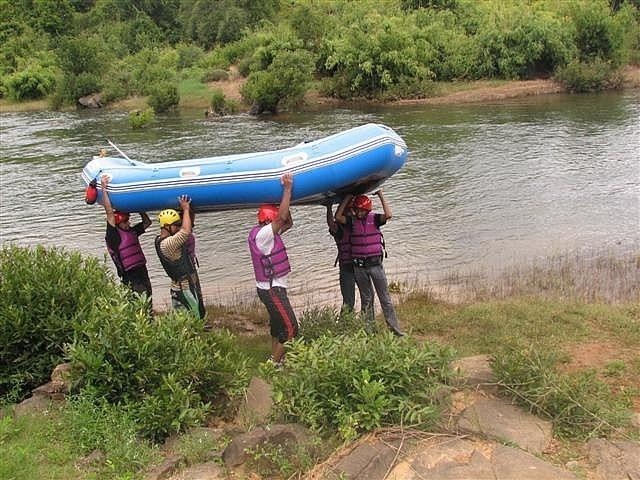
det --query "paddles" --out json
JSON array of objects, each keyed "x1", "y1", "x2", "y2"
[{"x1": 105, "y1": 138, "x2": 135, "y2": 165}]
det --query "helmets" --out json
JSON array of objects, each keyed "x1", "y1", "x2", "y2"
[
  {"x1": 353, "y1": 195, "x2": 372, "y2": 212},
  {"x1": 113, "y1": 211, "x2": 129, "y2": 224},
  {"x1": 257, "y1": 205, "x2": 279, "y2": 226},
  {"x1": 158, "y1": 209, "x2": 179, "y2": 228}
]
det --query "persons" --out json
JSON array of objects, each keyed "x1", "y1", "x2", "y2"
[
  {"x1": 101, "y1": 174, "x2": 152, "y2": 323},
  {"x1": 319, "y1": 195, "x2": 356, "y2": 320},
  {"x1": 248, "y1": 172, "x2": 298, "y2": 363},
  {"x1": 177, "y1": 206, "x2": 196, "y2": 266},
  {"x1": 155, "y1": 193, "x2": 213, "y2": 332},
  {"x1": 334, "y1": 187, "x2": 406, "y2": 337}
]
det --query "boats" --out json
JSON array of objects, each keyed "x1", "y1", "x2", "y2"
[{"x1": 78, "y1": 122, "x2": 409, "y2": 213}]
